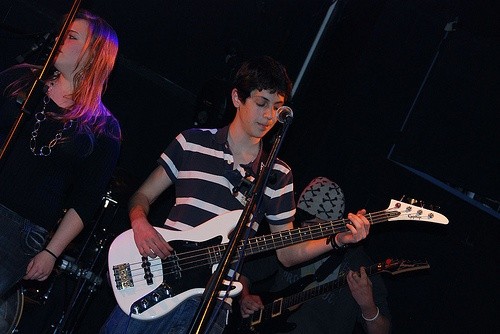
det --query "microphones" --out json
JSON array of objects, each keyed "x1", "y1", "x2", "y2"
[{"x1": 276, "y1": 106, "x2": 293, "y2": 124}]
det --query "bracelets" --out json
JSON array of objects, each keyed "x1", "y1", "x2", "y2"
[
  {"x1": 43, "y1": 248, "x2": 59, "y2": 261},
  {"x1": 326, "y1": 232, "x2": 347, "y2": 251},
  {"x1": 362, "y1": 305, "x2": 380, "y2": 321}
]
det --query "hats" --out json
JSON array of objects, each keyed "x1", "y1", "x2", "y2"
[{"x1": 297, "y1": 177, "x2": 345, "y2": 222}]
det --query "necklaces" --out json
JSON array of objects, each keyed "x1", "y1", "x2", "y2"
[{"x1": 29, "y1": 68, "x2": 82, "y2": 155}]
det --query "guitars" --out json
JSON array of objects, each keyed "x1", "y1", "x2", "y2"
[
  {"x1": 221, "y1": 257, "x2": 431, "y2": 334},
  {"x1": 107, "y1": 194, "x2": 449, "y2": 320}
]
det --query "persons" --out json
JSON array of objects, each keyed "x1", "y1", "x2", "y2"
[
  {"x1": 103, "y1": 54, "x2": 370, "y2": 334},
  {"x1": 237, "y1": 176, "x2": 391, "y2": 334},
  {"x1": 0, "y1": 9, "x2": 125, "y2": 312}
]
